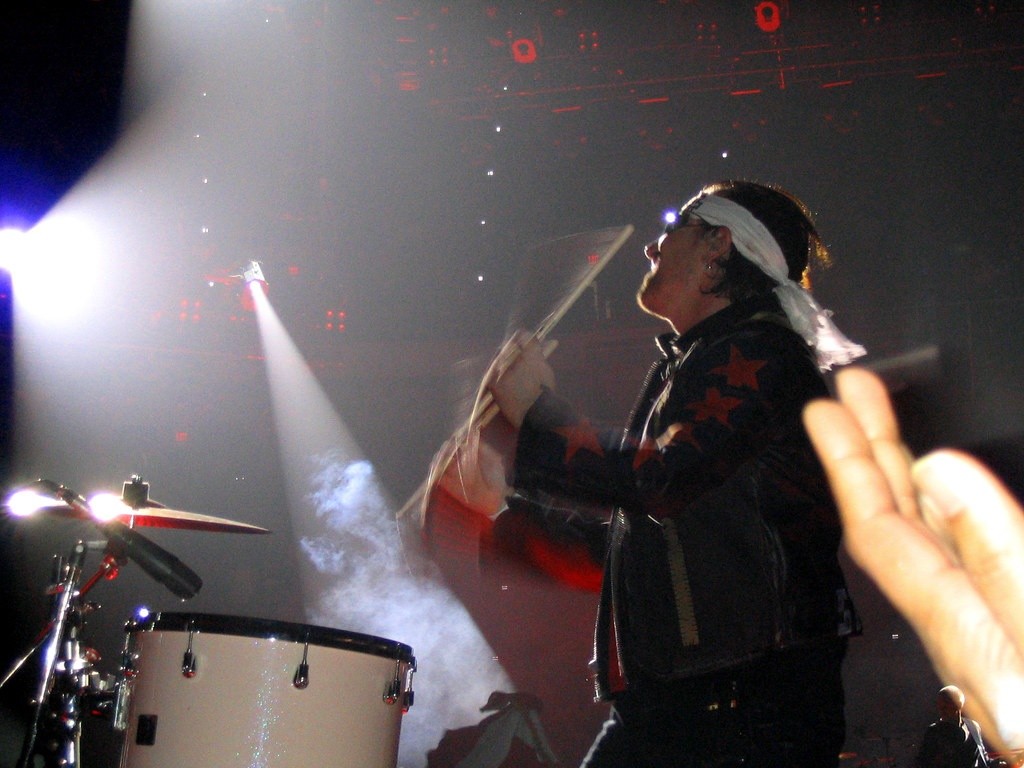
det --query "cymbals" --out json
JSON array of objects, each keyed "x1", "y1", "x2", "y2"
[{"x1": 15, "y1": 493, "x2": 274, "y2": 540}]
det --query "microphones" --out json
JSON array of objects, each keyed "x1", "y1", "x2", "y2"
[{"x1": 41, "y1": 478, "x2": 202, "y2": 600}]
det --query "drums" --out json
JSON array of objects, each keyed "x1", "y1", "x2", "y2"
[{"x1": 108, "y1": 604, "x2": 420, "y2": 768}]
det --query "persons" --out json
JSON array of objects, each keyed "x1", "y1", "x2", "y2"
[
  {"x1": 445, "y1": 183, "x2": 858, "y2": 768},
  {"x1": 919, "y1": 685, "x2": 1007, "y2": 768}
]
